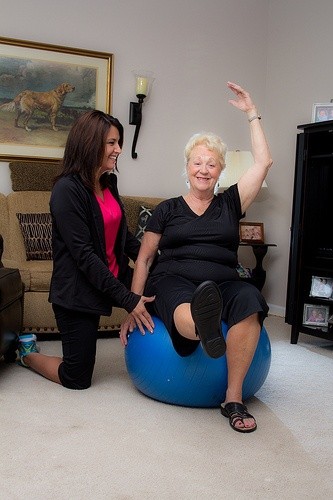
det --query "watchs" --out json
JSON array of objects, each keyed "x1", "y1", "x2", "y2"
[{"x1": 248, "y1": 112, "x2": 261, "y2": 122}]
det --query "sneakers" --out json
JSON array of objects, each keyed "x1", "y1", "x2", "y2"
[{"x1": 18, "y1": 334, "x2": 40, "y2": 366}]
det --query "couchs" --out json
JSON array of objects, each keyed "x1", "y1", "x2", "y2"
[{"x1": 0, "y1": 191, "x2": 170, "y2": 333}]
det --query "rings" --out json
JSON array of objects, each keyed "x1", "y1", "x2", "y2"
[{"x1": 119, "y1": 332, "x2": 121, "y2": 334}]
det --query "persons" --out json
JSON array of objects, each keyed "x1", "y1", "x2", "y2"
[
  {"x1": 307, "y1": 308, "x2": 325, "y2": 322},
  {"x1": 2, "y1": 110, "x2": 159, "y2": 390},
  {"x1": 313, "y1": 278, "x2": 331, "y2": 296},
  {"x1": 316, "y1": 109, "x2": 333, "y2": 120},
  {"x1": 242, "y1": 226, "x2": 260, "y2": 239},
  {"x1": 120, "y1": 81, "x2": 273, "y2": 434}
]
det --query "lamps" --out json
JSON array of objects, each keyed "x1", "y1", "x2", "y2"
[
  {"x1": 214, "y1": 150, "x2": 269, "y2": 189},
  {"x1": 128, "y1": 75, "x2": 148, "y2": 159}
]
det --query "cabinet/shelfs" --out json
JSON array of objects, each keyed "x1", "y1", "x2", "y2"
[{"x1": 285, "y1": 120, "x2": 333, "y2": 344}]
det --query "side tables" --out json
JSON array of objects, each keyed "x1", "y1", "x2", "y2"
[{"x1": 238, "y1": 244, "x2": 278, "y2": 292}]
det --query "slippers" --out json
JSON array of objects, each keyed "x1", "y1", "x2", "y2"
[
  {"x1": 220, "y1": 402, "x2": 257, "y2": 433},
  {"x1": 189, "y1": 280, "x2": 227, "y2": 359}
]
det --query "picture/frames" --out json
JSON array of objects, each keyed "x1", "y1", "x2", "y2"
[
  {"x1": 309, "y1": 276, "x2": 333, "y2": 299},
  {"x1": 303, "y1": 304, "x2": 328, "y2": 326},
  {"x1": 239, "y1": 222, "x2": 264, "y2": 244},
  {"x1": 312, "y1": 103, "x2": 333, "y2": 123},
  {"x1": 0, "y1": 36, "x2": 114, "y2": 161}
]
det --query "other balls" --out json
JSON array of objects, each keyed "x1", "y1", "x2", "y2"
[{"x1": 125, "y1": 312, "x2": 272, "y2": 407}]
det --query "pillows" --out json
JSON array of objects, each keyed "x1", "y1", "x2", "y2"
[{"x1": 16, "y1": 212, "x2": 54, "y2": 261}]
